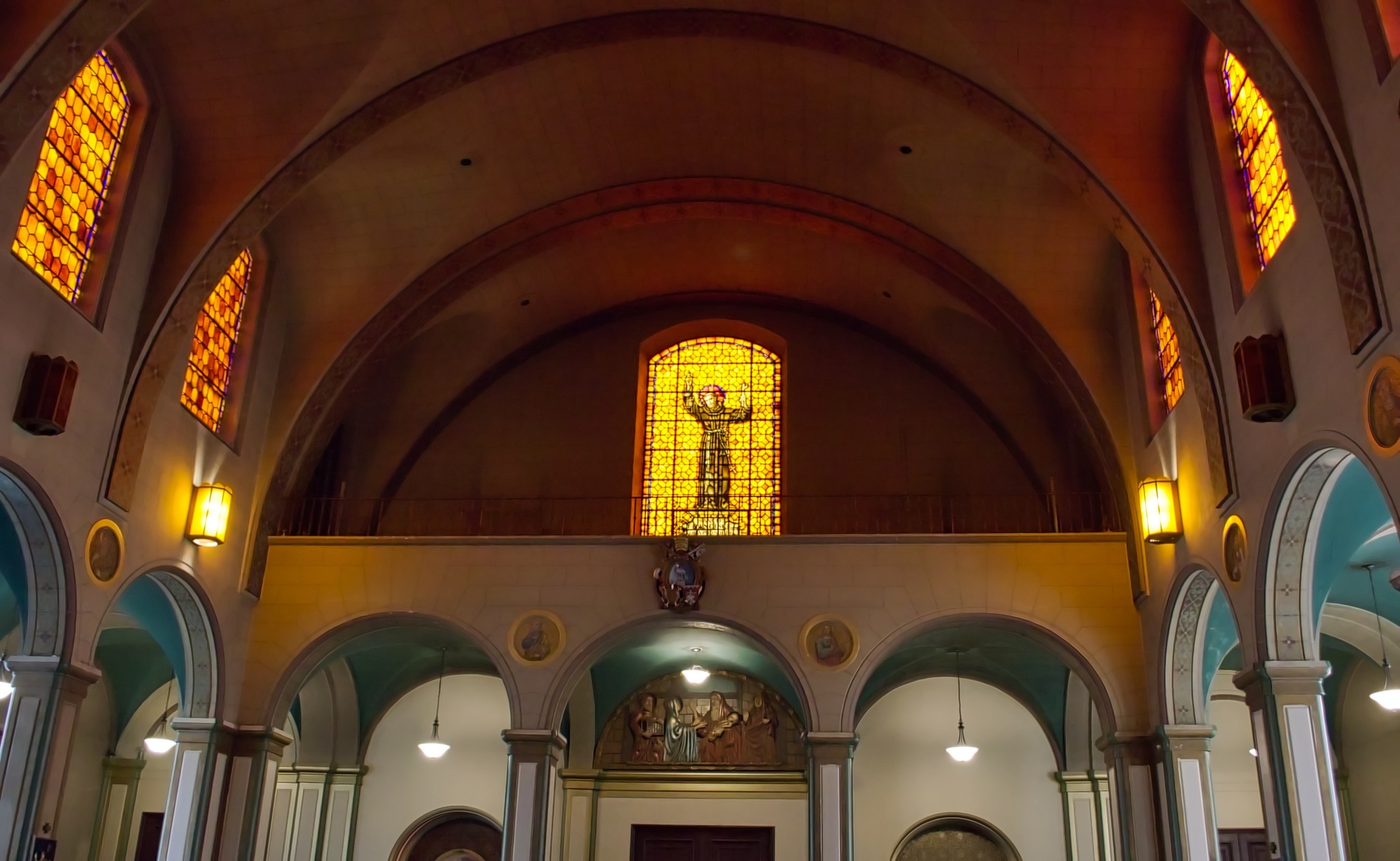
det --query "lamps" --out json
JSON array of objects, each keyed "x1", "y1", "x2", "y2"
[
  {"x1": 681, "y1": 648, "x2": 711, "y2": 685},
  {"x1": 417, "y1": 648, "x2": 451, "y2": 759},
  {"x1": 13, "y1": 353, "x2": 79, "y2": 436},
  {"x1": 144, "y1": 667, "x2": 177, "y2": 753},
  {"x1": 1138, "y1": 475, "x2": 1184, "y2": 545},
  {"x1": 945, "y1": 647, "x2": 979, "y2": 762},
  {"x1": 183, "y1": 482, "x2": 232, "y2": 547},
  {"x1": 0, "y1": 598, "x2": 19, "y2": 700},
  {"x1": 1360, "y1": 564, "x2": 1400, "y2": 711},
  {"x1": 1233, "y1": 333, "x2": 1296, "y2": 424}
]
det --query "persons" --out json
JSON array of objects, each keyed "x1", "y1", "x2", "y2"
[
  {"x1": 743, "y1": 692, "x2": 779, "y2": 763},
  {"x1": 522, "y1": 616, "x2": 550, "y2": 661},
  {"x1": 688, "y1": 691, "x2": 742, "y2": 762},
  {"x1": 815, "y1": 624, "x2": 844, "y2": 666},
  {"x1": 663, "y1": 695, "x2": 708, "y2": 762},
  {"x1": 682, "y1": 372, "x2": 750, "y2": 508},
  {"x1": 710, "y1": 711, "x2": 739, "y2": 735},
  {"x1": 628, "y1": 692, "x2": 664, "y2": 762}
]
